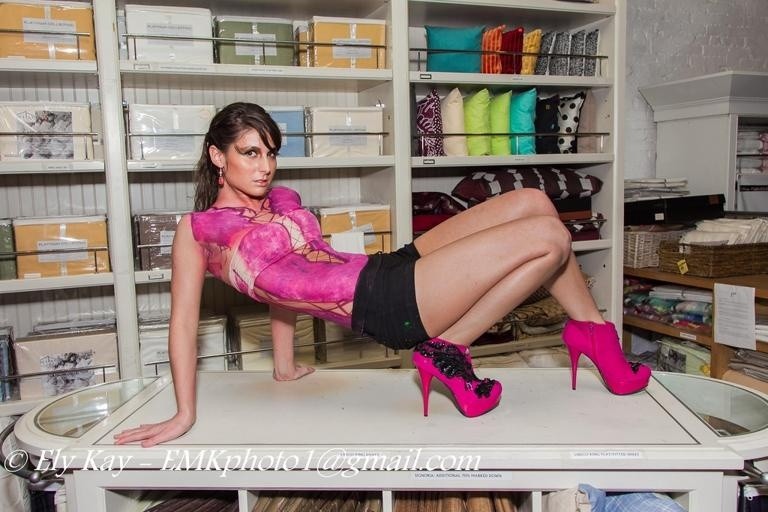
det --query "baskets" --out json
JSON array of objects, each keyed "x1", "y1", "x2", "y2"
[
  {"x1": 658, "y1": 239, "x2": 768, "y2": 279},
  {"x1": 625, "y1": 230, "x2": 687, "y2": 270}
]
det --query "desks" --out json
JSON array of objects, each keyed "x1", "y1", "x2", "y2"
[{"x1": 2, "y1": 372, "x2": 766, "y2": 511}]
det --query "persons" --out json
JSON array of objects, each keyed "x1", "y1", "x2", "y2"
[{"x1": 113, "y1": 103, "x2": 651, "y2": 448}]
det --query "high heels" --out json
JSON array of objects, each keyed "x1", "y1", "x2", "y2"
[
  {"x1": 412, "y1": 338, "x2": 503, "y2": 418},
  {"x1": 561, "y1": 318, "x2": 651, "y2": 395}
]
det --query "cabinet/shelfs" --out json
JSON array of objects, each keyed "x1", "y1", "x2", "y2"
[
  {"x1": 623, "y1": 266, "x2": 768, "y2": 397},
  {"x1": 0, "y1": 0, "x2": 627, "y2": 370},
  {"x1": 656, "y1": 117, "x2": 767, "y2": 215}
]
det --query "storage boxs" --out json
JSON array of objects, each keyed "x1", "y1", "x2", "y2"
[{"x1": 0, "y1": 2, "x2": 391, "y2": 400}]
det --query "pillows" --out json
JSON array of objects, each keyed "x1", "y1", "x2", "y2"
[{"x1": 412, "y1": 24, "x2": 608, "y2": 346}]
